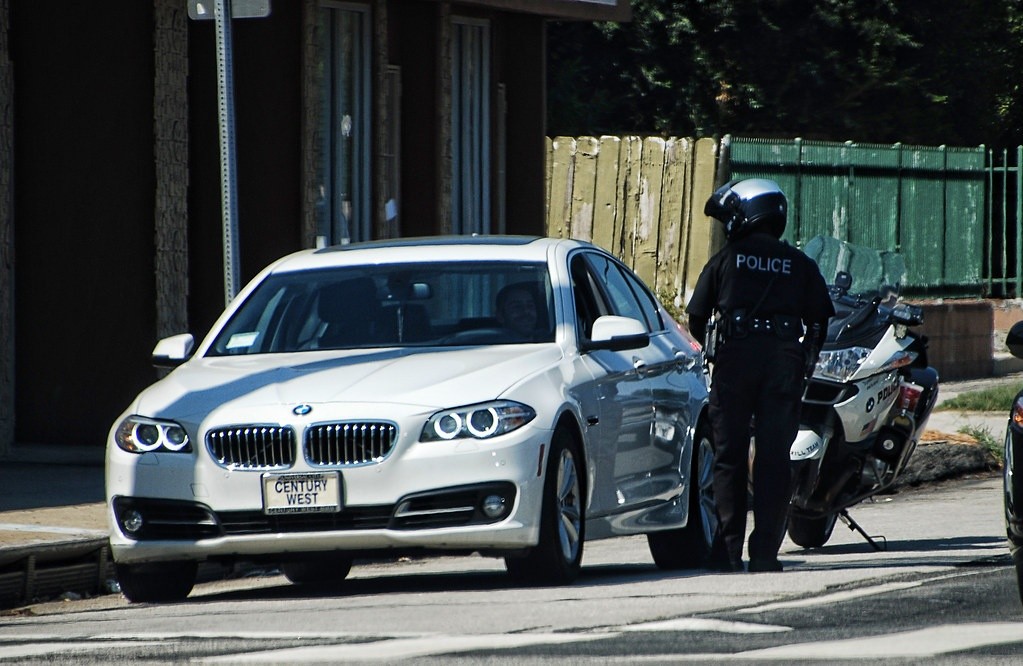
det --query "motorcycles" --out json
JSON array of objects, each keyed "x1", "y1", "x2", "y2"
[{"x1": 780, "y1": 233, "x2": 939, "y2": 552}]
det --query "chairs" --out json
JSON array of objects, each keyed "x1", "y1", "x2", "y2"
[{"x1": 321, "y1": 283, "x2": 431, "y2": 347}]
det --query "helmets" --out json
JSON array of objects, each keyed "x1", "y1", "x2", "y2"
[{"x1": 704, "y1": 179, "x2": 787, "y2": 244}]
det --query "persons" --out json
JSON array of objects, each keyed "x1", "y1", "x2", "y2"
[
  {"x1": 683, "y1": 178, "x2": 836, "y2": 572},
  {"x1": 495, "y1": 282, "x2": 537, "y2": 333}
]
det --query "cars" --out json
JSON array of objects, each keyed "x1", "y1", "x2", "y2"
[
  {"x1": 1002, "y1": 317, "x2": 1023, "y2": 601},
  {"x1": 106, "y1": 234, "x2": 715, "y2": 604}
]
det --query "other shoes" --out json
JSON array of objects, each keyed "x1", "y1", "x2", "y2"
[
  {"x1": 709, "y1": 557, "x2": 744, "y2": 573},
  {"x1": 747, "y1": 560, "x2": 784, "y2": 572}
]
